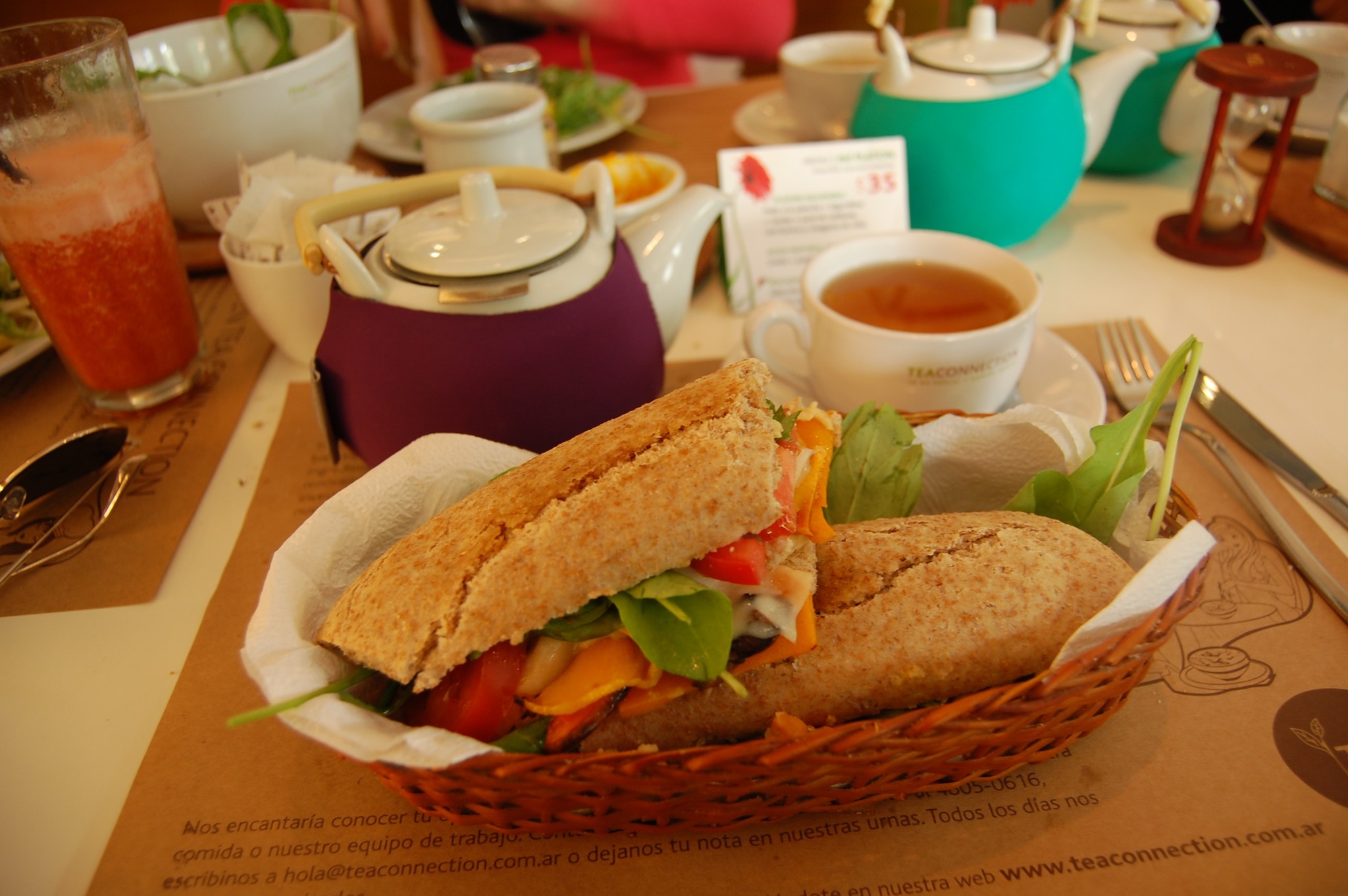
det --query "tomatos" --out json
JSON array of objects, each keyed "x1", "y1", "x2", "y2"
[
  {"x1": 390, "y1": 633, "x2": 533, "y2": 740},
  {"x1": 686, "y1": 441, "x2": 797, "y2": 583}
]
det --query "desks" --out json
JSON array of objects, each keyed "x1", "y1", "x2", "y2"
[{"x1": 0, "y1": 66, "x2": 1348, "y2": 896}]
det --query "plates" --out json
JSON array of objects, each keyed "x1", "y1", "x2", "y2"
[
  {"x1": 731, "y1": 91, "x2": 799, "y2": 148},
  {"x1": 0, "y1": 316, "x2": 52, "y2": 380},
  {"x1": 359, "y1": 67, "x2": 649, "y2": 163},
  {"x1": 733, "y1": 319, "x2": 1109, "y2": 439},
  {"x1": 1229, "y1": 100, "x2": 1330, "y2": 151}
]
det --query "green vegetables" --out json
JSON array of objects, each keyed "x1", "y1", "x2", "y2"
[
  {"x1": 225, "y1": 336, "x2": 1207, "y2": 743},
  {"x1": 0, "y1": 264, "x2": 43, "y2": 340},
  {"x1": 395, "y1": 38, "x2": 670, "y2": 138},
  {"x1": 47, "y1": 0, "x2": 301, "y2": 91}
]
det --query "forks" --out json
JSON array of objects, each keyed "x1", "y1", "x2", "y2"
[{"x1": 1091, "y1": 319, "x2": 1348, "y2": 623}]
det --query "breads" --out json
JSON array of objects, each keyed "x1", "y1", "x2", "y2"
[
  {"x1": 309, "y1": 353, "x2": 785, "y2": 693},
  {"x1": 551, "y1": 508, "x2": 1140, "y2": 757}
]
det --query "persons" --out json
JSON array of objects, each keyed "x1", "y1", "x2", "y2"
[{"x1": 415, "y1": 0, "x2": 799, "y2": 99}]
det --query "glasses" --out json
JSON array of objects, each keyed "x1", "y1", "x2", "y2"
[{"x1": 0, "y1": 423, "x2": 150, "y2": 588}]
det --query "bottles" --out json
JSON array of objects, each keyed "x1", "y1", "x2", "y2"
[
  {"x1": 471, "y1": 45, "x2": 541, "y2": 88},
  {"x1": 1314, "y1": 91, "x2": 1348, "y2": 211}
]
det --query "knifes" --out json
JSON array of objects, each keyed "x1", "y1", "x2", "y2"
[{"x1": 1175, "y1": 363, "x2": 1348, "y2": 525}]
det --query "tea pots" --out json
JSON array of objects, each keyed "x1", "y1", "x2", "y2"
[
  {"x1": 853, "y1": 5, "x2": 1154, "y2": 254},
  {"x1": 1039, "y1": 0, "x2": 1226, "y2": 178},
  {"x1": 289, "y1": 161, "x2": 731, "y2": 466}
]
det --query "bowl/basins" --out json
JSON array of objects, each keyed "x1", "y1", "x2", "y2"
[{"x1": 33, "y1": 9, "x2": 364, "y2": 237}]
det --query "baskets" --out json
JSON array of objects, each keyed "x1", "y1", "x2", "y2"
[{"x1": 365, "y1": 477, "x2": 1211, "y2": 837}]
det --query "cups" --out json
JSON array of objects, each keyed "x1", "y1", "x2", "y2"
[
  {"x1": 215, "y1": 202, "x2": 403, "y2": 367},
  {"x1": 745, "y1": 227, "x2": 1045, "y2": 426},
  {"x1": 0, "y1": 17, "x2": 203, "y2": 415},
  {"x1": 776, "y1": 29, "x2": 910, "y2": 140},
  {"x1": 406, "y1": 79, "x2": 553, "y2": 173},
  {"x1": 570, "y1": 150, "x2": 685, "y2": 228},
  {"x1": 1242, "y1": 19, "x2": 1348, "y2": 128}
]
